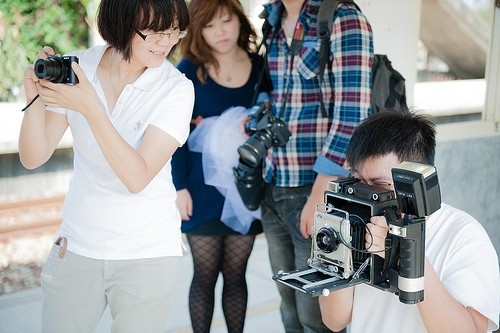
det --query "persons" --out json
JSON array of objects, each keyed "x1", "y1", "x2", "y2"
[
  {"x1": 175, "y1": 0, "x2": 274, "y2": 333},
  {"x1": 18, "y1": 0, "x2": 195, "y2": 333},
  {"x1": 318, "y1": 104, "x2": 500, "y2": 333},
  {"x1": 241, "y1": 0, "x2": 374, "y2": 333}
]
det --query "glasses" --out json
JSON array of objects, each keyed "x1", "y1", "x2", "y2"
[
  {"x1": 360, "y1": 180, "x2": 394, "y2": 190},
  {"x1": 135, "y1": 26, "x2": 189, "y2": 42}
]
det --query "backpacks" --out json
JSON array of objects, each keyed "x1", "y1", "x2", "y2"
[{"x1": 259, "y1": 0, "x2": 410, "y2": 118}]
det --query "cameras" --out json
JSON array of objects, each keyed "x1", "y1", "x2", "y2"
[
  {"x1": 271, "y1": 160, "x2": 442, "y2": 304},
  {"x1": 34, "y1": 55, "x2": 79, "y2": 86},
  {"x1": 236, "y1": 102, "x2": 289, "y2": 168}
]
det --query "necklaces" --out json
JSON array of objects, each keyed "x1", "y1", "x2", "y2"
[
  {"x1": 283, "y1": 23, "x2": 292, "y2": 46},
  {"x1": 224, "y1": 72, "x2": 232, "y2": 82}
]
